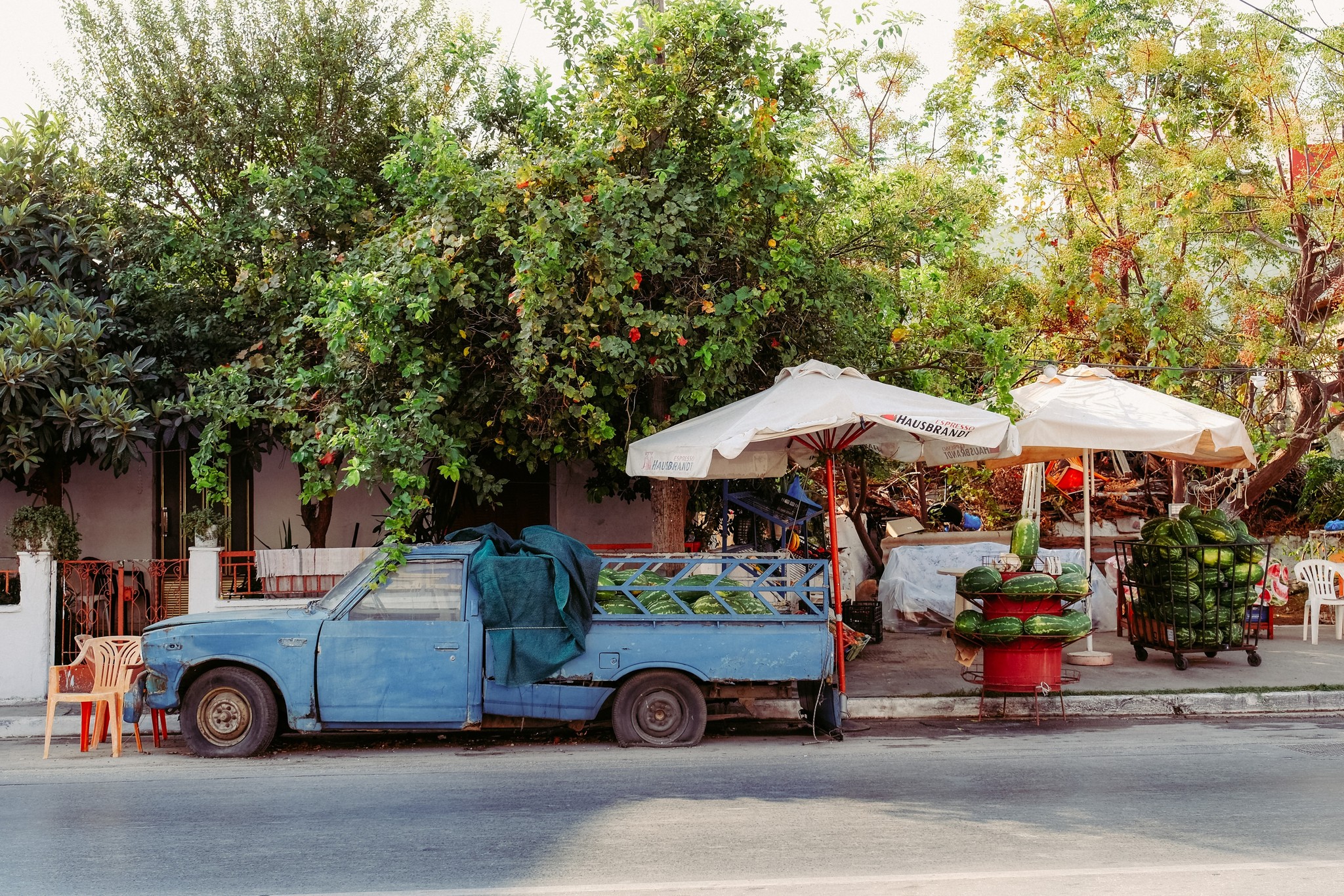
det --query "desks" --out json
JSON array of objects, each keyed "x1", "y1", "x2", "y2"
[
  {"x1": 1241, "y1": 604, "x2": 1271, "y2": 624},
  {"x1": 1308, "y1": 528, "x2": 1344, "y2": 561},
  {"x1": 935, "y1": 567, "x2": 974, "y2": 629},
  {"x1": 880, "y1": 529, "x2": 1013, "y2": 568}
]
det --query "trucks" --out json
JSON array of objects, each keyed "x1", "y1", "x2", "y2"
[{"x1": 121, "y1": 537, "x2": 847, "y2": 760}]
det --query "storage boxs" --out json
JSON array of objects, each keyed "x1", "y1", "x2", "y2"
[
  {"x1": 884, "y1": 516, "x2": 925, "y2": 539},
  {"x1": 810, "y1": 598, "x2": 884, "y2": 645}
]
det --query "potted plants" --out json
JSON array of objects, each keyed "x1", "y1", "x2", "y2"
[
  {"x1": 4, "y1": 501, "x2": 84, "y2": 565},
  {"x1": 178, "y1": 504, "x2": 235, "y2": 548}
]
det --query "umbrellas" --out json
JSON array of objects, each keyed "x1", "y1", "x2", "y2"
[
  {"x1": 969, "y1": 364, "x2": 1258, "y2": 650},
  {"x1": 626, "y1": 360, "x2": 1023, "y2": 720}
]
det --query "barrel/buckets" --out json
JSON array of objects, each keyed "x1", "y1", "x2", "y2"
[{"x1": 981, "y1": 571, "x2": 1063, "y2": 694}]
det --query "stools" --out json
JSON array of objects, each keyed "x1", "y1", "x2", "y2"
[{"x1": 71, "y1": 592, "x2": 111, "y2": 641}]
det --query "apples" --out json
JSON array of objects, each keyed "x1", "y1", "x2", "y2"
[{"x1": 794, "y1": 547, "x2": 831, "y2": 557}]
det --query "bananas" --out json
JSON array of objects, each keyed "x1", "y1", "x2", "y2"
[{"x1": 789, "y1": 531, "x2": 800, "y2": 551}]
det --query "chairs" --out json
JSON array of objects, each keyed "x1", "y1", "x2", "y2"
[
  {"x1": 1043, "y1": 455, "x2": 1111, "y2": 503},
  {"x1": 1294, "y1": 558, "x2": 1344, "y2": 645},
  {"x1": 42, "y1": 634, "x2": 168, "y2": 759},
  {"x1": 79, "y1": 556, "x2": 152, "y2": 637}
]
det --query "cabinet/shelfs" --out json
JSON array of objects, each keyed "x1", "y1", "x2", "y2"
[
  {"x1": 946, "y1": 550, "x2": 1100, "y2": 726},
  {"x1": 721, "y1": 478, "x2": 833, "y2": 604}
]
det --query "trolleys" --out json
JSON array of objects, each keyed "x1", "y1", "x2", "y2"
[{"x1": 1112, "y1": 538, "x2": 1273, "y2": 671}]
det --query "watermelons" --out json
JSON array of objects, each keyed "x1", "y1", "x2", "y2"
[
  {"x1": 955, "y1": 609, "x2": 1091, "y2": 643},
  {"x1": 596, "y1": 568, "x2": 771, "y2": 615},
  {"x1": 1009, "y1": 517, "x2": 1039, "y2": 572},
  {"x1": 957, "y1": 562, "x2": 1089, "y2": 602},
  {"x1": 1124, "y1": 504, "x2": 1265, "y2": 647}
]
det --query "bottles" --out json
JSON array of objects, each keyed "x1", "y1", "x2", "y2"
[{"x1": 688, "y1": 531, "x2": 695, "y2": 542}]
